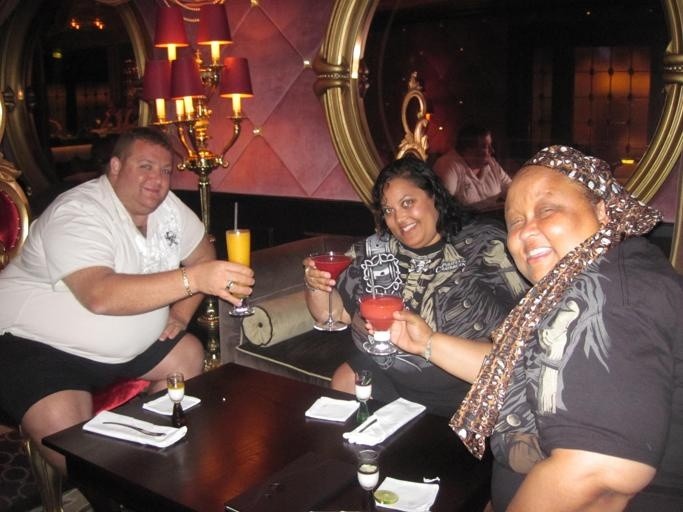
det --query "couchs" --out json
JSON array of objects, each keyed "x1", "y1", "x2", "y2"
[{"x1": 214, "y1": 232, "x2": 360, "y2": 417}]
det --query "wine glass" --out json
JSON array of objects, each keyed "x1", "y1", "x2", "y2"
[
  {"x1": 355, "y1": 369, "x2": 372, "y2": 423},
  {"x1": 225, "y1": 228, "x2": 254, "y2": 317},
  {"x1": 357, "y1": 294, "x2": 405, "y2": 356},
  {"x1": 166, "y1": 371, "x2": 188, "y2": 428},
  {"x1": 307, "y1": 251, "x2": 354, "y2": 333},
  {"x1": 356, "y1": 449, "x2": 380, "y2": 512}
]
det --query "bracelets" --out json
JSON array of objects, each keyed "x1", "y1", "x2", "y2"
[
  {"x1": 304, "y1": 277, "x2": 318, "y2": 292},
  {"x1": 425, "y1": 331, "x2": 436, "y2": 361},
  {"x1": 180, "y1": 266, "x2": 193, "y2": 297}
]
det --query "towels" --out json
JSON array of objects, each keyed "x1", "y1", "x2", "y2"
[
  {"x1": 82, "y1": 408, "x2": 187, "y2": 449},
  {"x1": 305, "y1": 397, "x2": 359, "y2": 423},
  {"x1": 142, "y1": 392, "x2": 200, "y2": 414},
  {"x1": 375, "y1": 476, "x2": 440, "y2": 512},
  {"x1": 342, "y1": 396, "x2": 427, "y2": 447}
]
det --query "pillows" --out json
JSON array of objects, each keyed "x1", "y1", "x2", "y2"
[{"x1": 242, "y1": 289, "x2": 321, "y2": 346}]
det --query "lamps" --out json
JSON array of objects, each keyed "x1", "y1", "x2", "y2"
[{"x1": 142, "y1": 3, "x2": 254, "y2": 329}]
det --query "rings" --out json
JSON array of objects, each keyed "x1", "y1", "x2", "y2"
[{"x1": 226, "y1": 280, "x2": 234, "y2": 289}]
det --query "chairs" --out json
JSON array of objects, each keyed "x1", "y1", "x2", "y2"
[{"x1": 0, "y1": 160, "x2": 149, "y2": 512}]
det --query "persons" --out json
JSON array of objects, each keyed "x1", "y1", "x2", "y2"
[
  {"x1": 366, "y1": 145, "x2": 682, "y2": 512},
  {"x1": 0, "y1": 129, "x2": 255, "y2": 478},
  {"x1": 299, "y1": 161, "x2": 530, "y2": 418},
  {"x1": 433, "y1": 124, "x2": 514, "y2": 206},
  {"x1": 90, "y1": 94, "x2": 140, "y2": 137}
]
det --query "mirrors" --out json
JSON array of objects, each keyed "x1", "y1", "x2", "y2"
[
  {"x1": 312, "y1": 0, "x2": 683, "y2": 220},
  {"x1": 0, "y1": 1, "x2": 148, "y2": 199}
]
